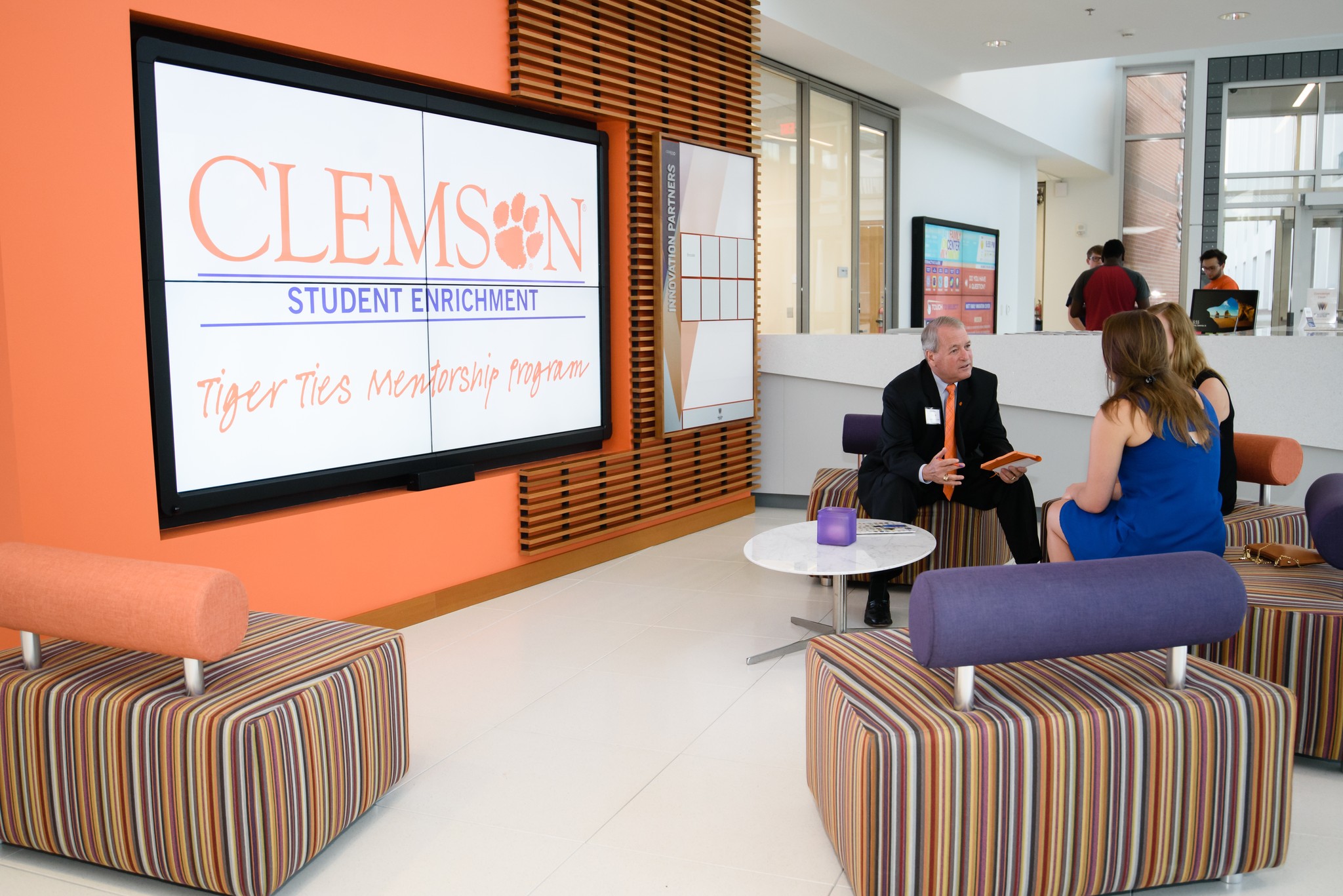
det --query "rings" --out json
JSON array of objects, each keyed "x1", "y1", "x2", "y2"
[
  {"x1": 943, "y1": 474, "x2": 950, "y2": 481},
  {"x1": 1009, "y1": 475, "x2": 1016, "y2": 481}
]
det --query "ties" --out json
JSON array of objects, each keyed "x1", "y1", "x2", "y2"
[{"x1": 942, "y1": 384, "x2": 957, "y2": 501}]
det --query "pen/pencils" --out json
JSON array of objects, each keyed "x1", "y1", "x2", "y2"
[{"x1": 936, "y1": 457, "x2": 964, "y2": 468}]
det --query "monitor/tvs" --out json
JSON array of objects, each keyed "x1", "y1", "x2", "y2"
[
  {"x1": 1189, "y1": 288, "x2": 1261, "y2": 336},
  {"x1": 911, "y1": 216, "x2": 1000, "y2": 334},
  {"x1": 133, "y1": 35, "x2": 616, "y2": 521}
]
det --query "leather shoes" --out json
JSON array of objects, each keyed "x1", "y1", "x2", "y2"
[{"x1": 863, "y1": 589, "x2": 892, "y2": 627}]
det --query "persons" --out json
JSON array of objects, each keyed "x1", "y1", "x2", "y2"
[
  {"x1": 1069, "y1": 238, "x2": 1152, "y2": 331},
  {"x1": 1065, "y1": 245, "x2": 1105, "y2": 330},
  {"x1": 1145, "y1": 302, "x2": 1238, "y2": 516},
  {"x1": 1046, "y1": 310, "x2": 1227, "y2": 565},
  {"x1": 1199, "y1": 249, "x2": 1241, "y2": 289},
  {"x1": 858, "y1": 314, "x2": 1043, "y2": 629}
]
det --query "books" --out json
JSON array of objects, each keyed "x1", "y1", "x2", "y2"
[
  {"x1": 856, "y1": 522, "x2": 916, "y2": 534},
  {"x1": 981, "y1": 451, "x2": 1042, "y2": 474}
]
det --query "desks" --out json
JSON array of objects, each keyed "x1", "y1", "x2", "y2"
[{"x1": 742, "y1": 515, "x2": 936, "y2": 670}]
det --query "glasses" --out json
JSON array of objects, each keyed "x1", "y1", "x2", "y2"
[
  {"x1": 1201, "y1": 265, "x2": 1219, "y2": 272},
  {"x1": 1089, "y1": 256, "x2": 1103, "y2": 262}
]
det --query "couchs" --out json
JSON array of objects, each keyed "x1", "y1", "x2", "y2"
[
  {"x1": 803, "y1": 546, "x2": 1299, "y2": 896},
  {"x1": 810, "y1": 410, "x2": 1022, "y2": 584},
  {"x1": 1215, "y1": 429, "x2": 1318, "y2": 560},
  {"x1": 0, "y1": 536, "x2": 406, "y2": 896},
  {"x1": 1191, "y1": 473, "x2": 1342, "y2": 763}
]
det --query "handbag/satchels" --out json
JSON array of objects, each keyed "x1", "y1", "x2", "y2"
[{"x1": 1245, "y1": 542, "x2": 1326, "y2": 566}]
